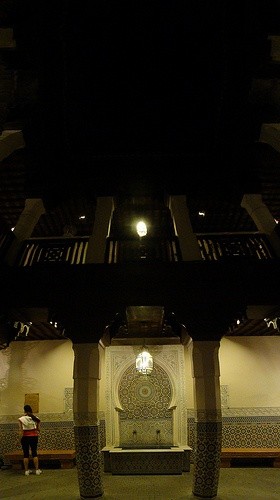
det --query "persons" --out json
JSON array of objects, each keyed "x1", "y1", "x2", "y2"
[{"x1": 17, "y1": 404, "x2": 43, "y2": 476}]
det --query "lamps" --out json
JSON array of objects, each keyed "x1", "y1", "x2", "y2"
[{"x1": 135, "y1": 345, "x2": 154, "y2": 376}]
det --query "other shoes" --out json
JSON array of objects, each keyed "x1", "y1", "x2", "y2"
[
  {"x1": 24, "y1": 470, "x2": 33, "y2": 476},
  {"x1": 35, "y1": 469, "x2": 42, "y2": 476}
]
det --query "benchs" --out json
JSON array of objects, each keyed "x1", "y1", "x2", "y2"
[
  {"x1": 220, "y1": 447, "x2": 280, "y2": 468},
  {"x1": 3, "y1": 449, "x2": 76, "y2": 469}
]
